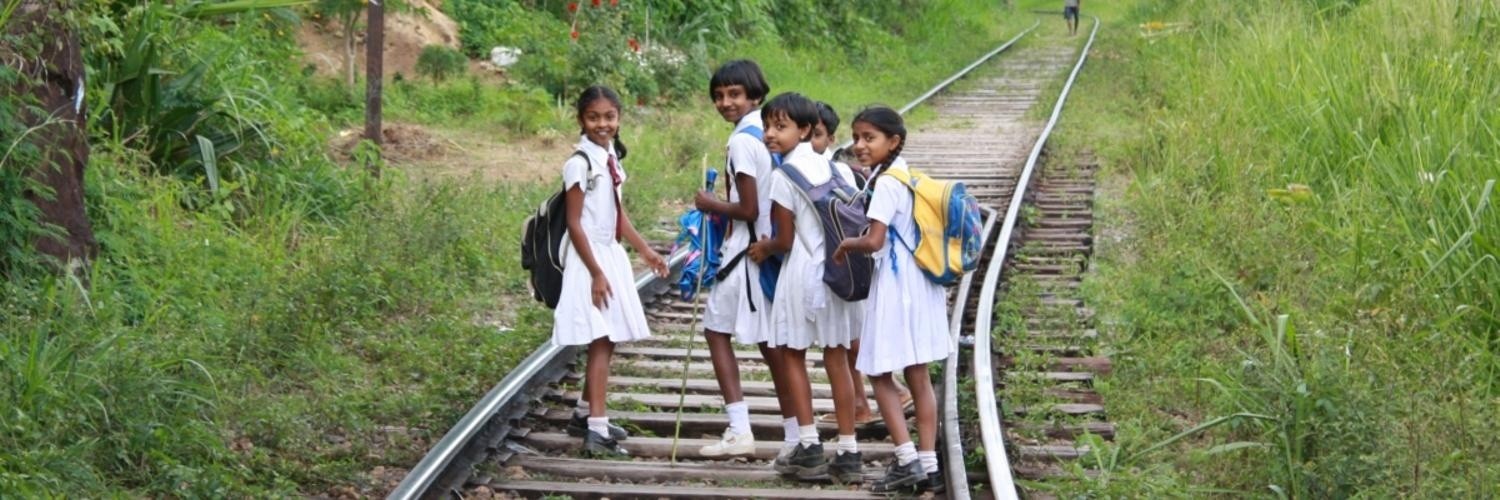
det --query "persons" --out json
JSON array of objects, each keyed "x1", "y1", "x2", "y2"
[
  {"x1": 1064, "y1": 0, "x2": 1081, "y2": 36},
  {"x1": 746, "y1": 91, "x2": 865, "y2": 489},
  {"x1": 831, "y1": 101, "x2": 957, "y2": 494},
  {"x1": 549, "y1": 81, "x2": 674, "y2": 461},
  {"x1": 806, "y1": 100, "x2": 919, "y2": 429},
  {"x1": 693, "y1": 60, "x2": 798, "y2": 463}
]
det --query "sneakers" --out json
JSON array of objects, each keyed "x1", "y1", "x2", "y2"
[
  {"x1": 699, "y1": 427, "x2": 756, "y2": 459},
  {"x1": 567, "y1": 409, "x2": 627, "y2": 440},
  {"x1": 769, "y1": 383, "x2": 946, "y2": 493},
  {"x1": 581, "y1": 428, "x2": 628, "y2": 457}
]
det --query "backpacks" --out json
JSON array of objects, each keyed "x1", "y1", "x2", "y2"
[
  {"x1": 876, "y1": 167, "x2": 983, "y2": 284},
  {"x1": 729, "y1": 126, "x2": 784, "y2": 304},
  {"x1": 521, "y1": 150, "x2": 591, "y2": 309},
  {"x1": 779, "y1": 160, "x2": 874, "y2": 301}
]
